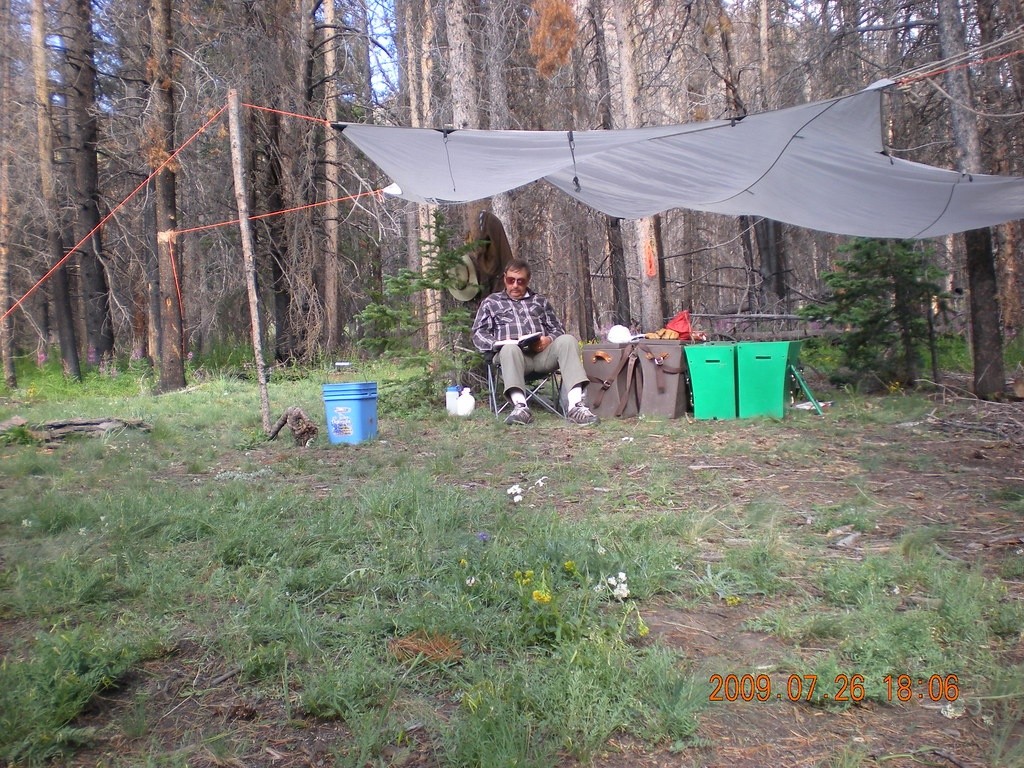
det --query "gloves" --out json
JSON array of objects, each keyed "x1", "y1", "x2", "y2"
[{"x1": 645, "y1": 327, "x2": 680, "y2": 339}]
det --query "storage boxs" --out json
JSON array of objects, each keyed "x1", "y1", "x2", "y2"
[
  {"x1": 582, "y1": 339, "x2": 804, "y2": 421},
  {"x1": 323, "y1": 382, "x2": 378, "y2": 446}
]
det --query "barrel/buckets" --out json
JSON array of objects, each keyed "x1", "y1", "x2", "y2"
[{"x1": 323, "y1": 361, "x2": 376, "y2": 446}]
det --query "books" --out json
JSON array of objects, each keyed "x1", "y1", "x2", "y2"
[{"x1": 493, "y1": 332, "x2": 541, "y2": 352}]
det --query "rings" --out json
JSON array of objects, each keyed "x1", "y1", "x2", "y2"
[{"x1": 539, "y1": 347, "x2": 540, "y2": 348}]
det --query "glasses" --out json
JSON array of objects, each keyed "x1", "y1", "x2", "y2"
[{"x1": 504, "y1": 272, "x2": 531, "y2": 285}]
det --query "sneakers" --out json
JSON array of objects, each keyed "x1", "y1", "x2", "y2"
[
  {"x1": 566, "y1": 402, "x2": 601, "y2": 429},
  {"x1": 504, "y1": 402, "x2": 535, "y2": 426}
]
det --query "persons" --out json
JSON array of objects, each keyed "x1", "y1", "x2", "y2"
[{"x1": 471, "y1": 259, "x2": 600, "y2": 426}]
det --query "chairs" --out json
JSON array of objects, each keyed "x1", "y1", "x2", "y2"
[{"x1": 483, "y1": 351, "x2": 562, "y2": 420}]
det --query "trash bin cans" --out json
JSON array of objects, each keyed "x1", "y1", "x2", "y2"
[{"x1": 323, "y1": 381, "x2": 379, "y2": 446}]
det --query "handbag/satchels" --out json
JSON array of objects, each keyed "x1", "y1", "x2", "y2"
[{"x1": 666, "y1": 310, "x2": 694, "y2": 340}]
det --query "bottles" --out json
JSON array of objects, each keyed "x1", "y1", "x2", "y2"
[
  {"x1": 457, "y1": 388, "x2": 476, "y2": 416},
  {"x1": 446, "y1": 384, "x2": 460, "y2": 413}
]
